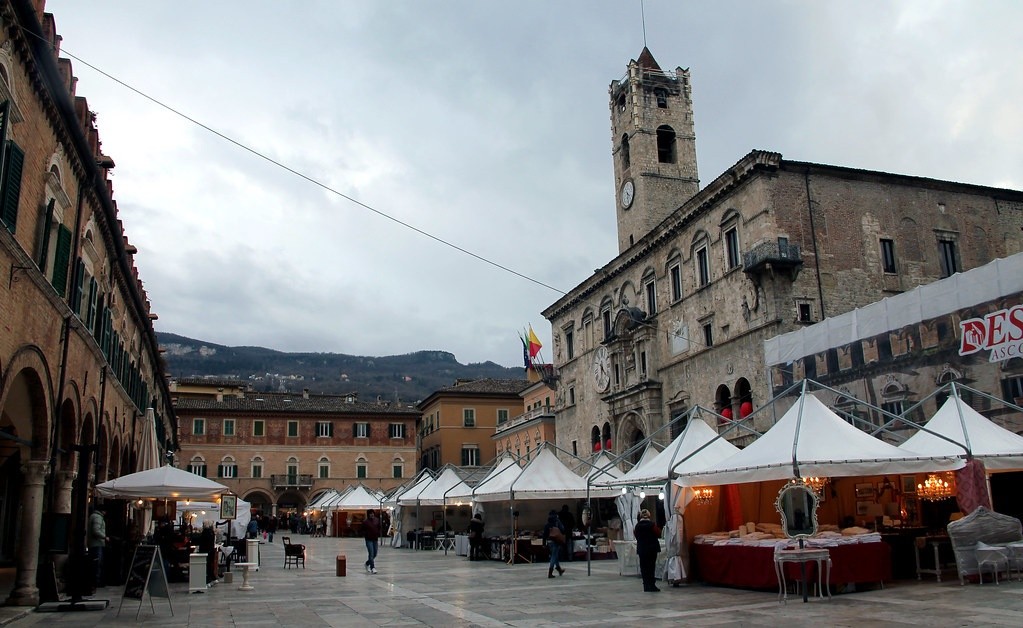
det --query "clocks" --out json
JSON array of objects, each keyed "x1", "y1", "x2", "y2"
[
  {"x1": 620, "y1": 178, "x2": 633, "y2": 209},
  {"x1": 592, "y1": 348, "x2": 610, "y2": 388}
]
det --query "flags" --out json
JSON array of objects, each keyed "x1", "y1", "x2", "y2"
[
  {"x1": 523, "y1": 330, "x2": 532, "y2": 361},
  {"x1": 519, "y1": 337, "x2": 530, "y2": 368},
  {"x1": 529, "y1": 327, "x2": 542, "y2": 358}
]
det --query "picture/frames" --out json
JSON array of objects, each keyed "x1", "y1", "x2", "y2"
[
  {"x1": 900, "y1": 475, "x2": 918, "y2": 494},
  {"x1": 856, "y1": 500, "x2": 874, "y2": 516},
  {"x1": 220, "y1": 494, "x2": 238, "y2": 519},
  {"x1": 856, "y1": 482, "x2": 874, "y2": 497},
  {"x1": 433, "y1": 511, "x2": 444, "y2": 520},
  {"x1": 877, "y1": 481, "x2": 895, "y2": 493}
]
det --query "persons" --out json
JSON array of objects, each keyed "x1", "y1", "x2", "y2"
[
  {"x1": 466, "y1": 513, "x2": 485, "y2": 560},
  {"x1": 557, "y1": 503, "x2": 576, "y2": 563},
  {"x1": 382, "y1": 511, "x2": 389, "y2": 537},
  {"x1": 247, "y1": 507, "x2": 327, "y2": 542},
  {"x1": 361, "y1": 509, "x2": 380, "y2": 575},
  {"x1": 438, "y1": 520, "x2": 453, "y2": 533},
  {"x1": 542, "y1": 510, "x2": 564, "y2": 578},
  {"x1": 632, "y1": 508, "x2": 660, "y2": 593},
  {"x1": 86, "y1": 502, "x2": 110, "y2": 596},
  {"x1": 189, "y1": 518, "x2": 219, "y2": 587}
]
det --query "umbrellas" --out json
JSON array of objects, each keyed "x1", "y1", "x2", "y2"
[
  {"x1": 94, "y1": 459, "x2": 227, "y2": 517},
  {"x1": 134, "y1": 406, "x2": 163, "y2": 534}
]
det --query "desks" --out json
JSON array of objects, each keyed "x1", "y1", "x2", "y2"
[
  {"x1": 435, "y1": 538, "x2": 455, "y2": 551},
  {"x1": 234, "y1": 563, "x2": 258, "y2": 590},
  {"x1": 976, "y1": 545, "x2": 1010, "y2": 586},
  {"x1": 885, "y1": 526, "x2": 929, "y2": 578},
  {"x1": 690, "y1": 541, "x2": 892, "y2": 597},
  {"x1": 773, "y1": 548, "x2": 833, "y2": 602},
  {"x1": 913, "y1": 533, "x2": 956, "y2": 582},
  {"x1": 407, "y1": 531, "x2": 443, "y2": 549}
]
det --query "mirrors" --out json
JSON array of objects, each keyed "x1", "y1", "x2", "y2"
[{"x1": 774, "y1": 483, "x2": 820, "y2": 536}]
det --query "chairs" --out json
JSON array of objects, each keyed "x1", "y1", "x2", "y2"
[{"x1": 282, "y1": 536, "x2": 306, "y2": 569}]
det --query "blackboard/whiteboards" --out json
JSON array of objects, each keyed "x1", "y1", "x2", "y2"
[{"x1": 124, "y1": 545, "x2": 171, "y2": 600}]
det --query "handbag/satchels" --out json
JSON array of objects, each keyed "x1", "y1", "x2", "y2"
[
  {"x1": 263, "y1": 529, "x2": 266, "y2": 539},
  {"x1": 246, "y1": 532, "x2": 250, "y2": 538},
  {"x1": 549, "y1": 521, "x2": 563, "y2": 543},
  {"x1": 468, "y1": 532, "x2": 475, "y2": 539}
]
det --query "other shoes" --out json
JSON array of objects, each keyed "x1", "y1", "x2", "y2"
[
  {"x1": 372, "y1": 568, "x2": 378, "y2": 573},
  {"x1": 364, "y1": 561, "x2": 370, "y2": 572},
  {"x1": 475, "y1": 558, "x2": 481, "y2": 560},
  {"x1": 470, "y1": 557, "x2": 475, "y2": 560},
  {"x1": 646, "y1": 586, "x2": 660, "y2": 591}
]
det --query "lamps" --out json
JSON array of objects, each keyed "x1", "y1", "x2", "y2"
[
  {"x1": 868, "y1": 505, "x2": 884, "y2": 532},
  {"x1": 918, "y1": 472, "x2": 952, "y2": 501},
  {"x1": 692, "y1": 487, "x2": 715, "y2": 507}
]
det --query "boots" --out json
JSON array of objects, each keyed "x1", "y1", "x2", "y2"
[
  {"x1": 555, "y1": 565, "x2": 565, "y2": 576},
  {"x1": 548, "y1": 568, "x2": 555, "y2": 578}
]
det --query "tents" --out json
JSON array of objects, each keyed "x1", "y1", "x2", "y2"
[
  {"x1": 304, "y1": 375, "x2": 1023, "y2": 587},
  {"x1": 175, "y1": 487, "x2": 252, "y2": 539}
]
